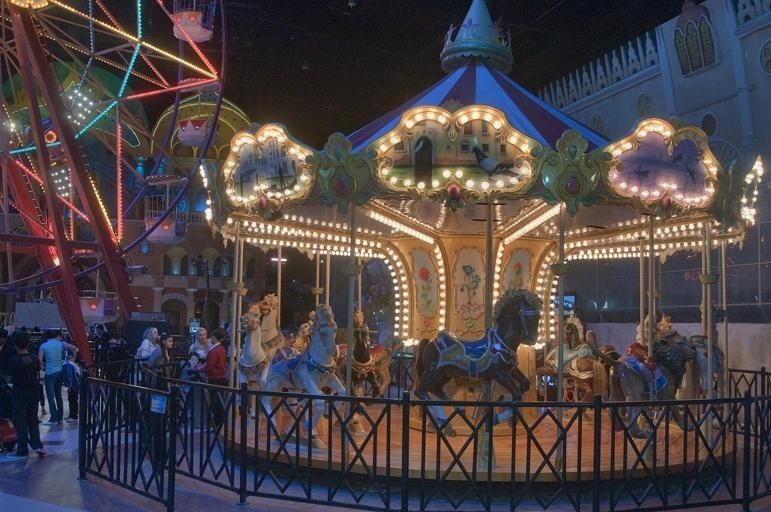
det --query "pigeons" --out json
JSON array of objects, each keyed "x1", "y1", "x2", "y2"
[{"x1": 472, "y1": 146, "x2": 519, "y2": 180}]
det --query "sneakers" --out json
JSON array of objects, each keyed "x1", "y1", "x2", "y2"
[
  {"x1": 42, "y1": 409, "x2": 78, "y2": 425},
  {"x1": 6, "y1": 449, "x2": 46, "y2": 459}
]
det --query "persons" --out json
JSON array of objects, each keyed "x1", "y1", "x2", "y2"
[{"x1": 0, "y1": 323, "x2": 227, "y2": 458}]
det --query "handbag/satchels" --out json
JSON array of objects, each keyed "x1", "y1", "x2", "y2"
[{"x1": 64, "y1": 359, "x2": 81, "y2": 388}]
